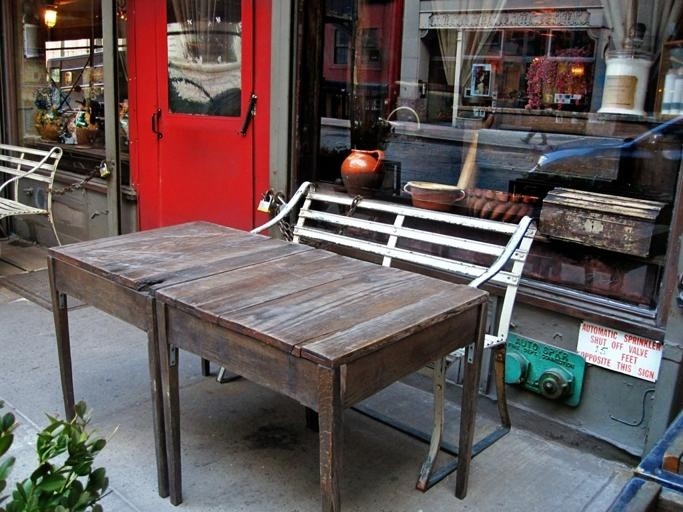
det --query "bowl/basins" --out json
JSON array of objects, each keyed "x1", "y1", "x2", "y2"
[{"x1": 404, "y1": 180, "x2": 466, "y2": 212}]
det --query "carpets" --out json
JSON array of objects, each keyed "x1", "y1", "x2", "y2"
[{"x1": 0, "y1": 267, "x2": 89, "y2": 312}]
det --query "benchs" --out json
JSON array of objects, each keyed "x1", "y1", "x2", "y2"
[
  {"x1": 214, "y1": 182, "x2": 539, "y2": 493},
  {"x1": 0, "y1": 144, "x2": 64, "y2": 246}
]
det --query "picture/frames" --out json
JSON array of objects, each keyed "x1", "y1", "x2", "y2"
[{"x1": 653, "y1": 39, "x2": 683, "y2": 122}]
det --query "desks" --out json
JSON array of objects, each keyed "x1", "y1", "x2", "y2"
[
  {"x1": 47, "y1": 219, "x2": 316, "y2": 490},
  {"x1": 154, "y1": 247, "x2": 489, "y2": 509}
]
